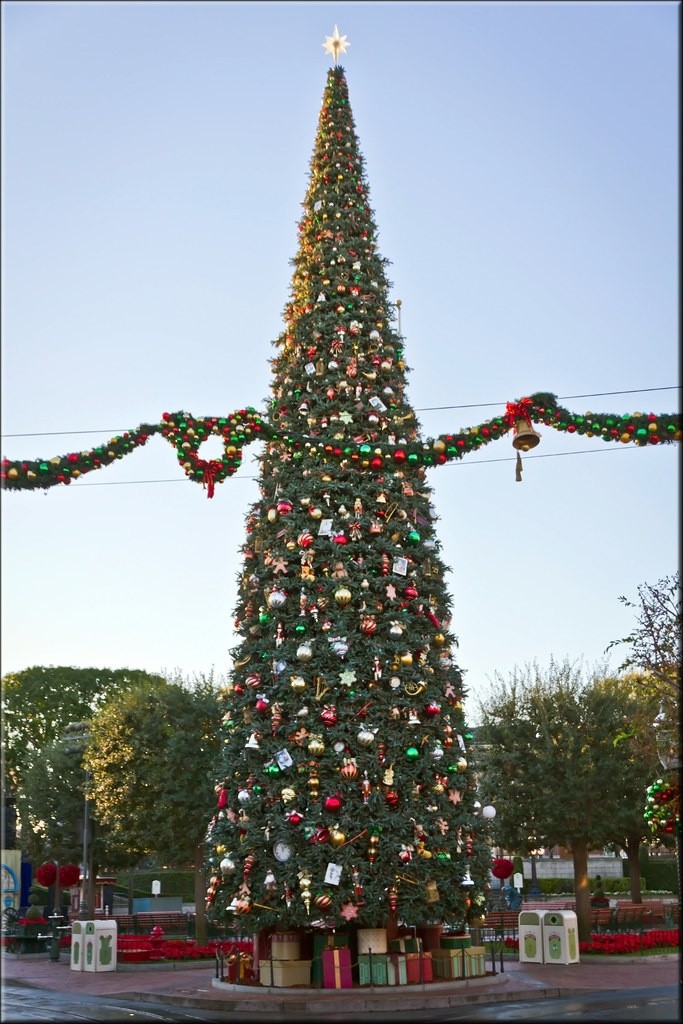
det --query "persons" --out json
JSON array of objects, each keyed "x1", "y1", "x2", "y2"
[
  {"x1": 589, "y1": 890, "x2": 610, "y2": 906},
  {"x1": 501, "y1": 886, "x2": 523, "y2": 910}
]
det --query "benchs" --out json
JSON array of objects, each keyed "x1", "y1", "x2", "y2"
[
  {"x1": 70, "y1": 913, "x2": 191, "y2": 935},
  {"x1": 488, "y1": 900, "x2": 681, "y2": 942}
]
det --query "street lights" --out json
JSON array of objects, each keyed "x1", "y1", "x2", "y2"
[{"x1": 481, "y1": 805, "x2": 505, "y2": 971}]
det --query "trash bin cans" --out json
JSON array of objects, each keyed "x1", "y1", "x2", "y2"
[
  {"x1": 70, "y1": 921, "x2": 88, "y2": 971},
  {"x1": 518, "y1": 910, "x2": 550, "y2": 964},
  {"x1": 83, "y1": 920, "x2": 117, "y2": 973},
  {"x1": 543, "y1": 909, "x2": 581, "y2": 965}
]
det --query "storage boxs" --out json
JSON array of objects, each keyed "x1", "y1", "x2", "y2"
[
  {"x1": 259, "y1": 926, "x2": 486, "y2": 989},
  {"x1": 229, "y1": 953, "x2": 251, "y2": 982}
]
